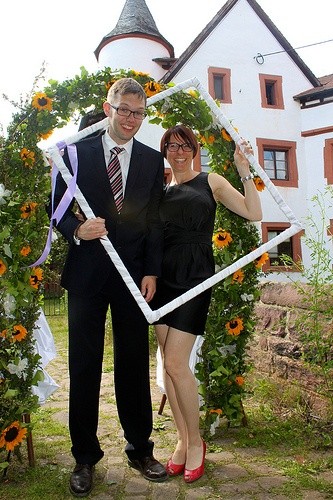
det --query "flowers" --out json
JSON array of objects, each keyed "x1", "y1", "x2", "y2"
[{"x1": 0, "y1": 65, "x2": 270, "y2": 477}]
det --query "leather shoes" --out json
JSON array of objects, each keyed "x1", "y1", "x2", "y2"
[
  {"x1": 127, "y1": 451, "x2": 166, "y2": 481},
  {"x1": 70, "y1": 466, "x2": 94, "y2": 493}
]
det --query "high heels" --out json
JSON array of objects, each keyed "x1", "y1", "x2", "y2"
[{"x1": 167, "y1": 440, "x2": 206, "y2": 482}]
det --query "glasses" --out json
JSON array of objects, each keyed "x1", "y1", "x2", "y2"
[
  {"x1": 166, "y1": 143, "x2": 192, "y2": 152},
  {"x1": 108, "y1": 102, "x2": 146, "y2": 118}
]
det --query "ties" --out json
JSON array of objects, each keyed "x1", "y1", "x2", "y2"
[{"x1": 107, "y1": 145, "x2": 126, "y2": 213}]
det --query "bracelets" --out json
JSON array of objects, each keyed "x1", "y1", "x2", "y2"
[{"x1": 241, "y1": 173, "x2": 254, "y2": 183}]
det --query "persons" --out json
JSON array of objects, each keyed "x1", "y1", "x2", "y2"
[
  {"x1": 46, "y1": 78, "x2": 165, "y2": 496},
  {"x1": 153, "y1": 123, "x2": 263, "y2": 483}
]
